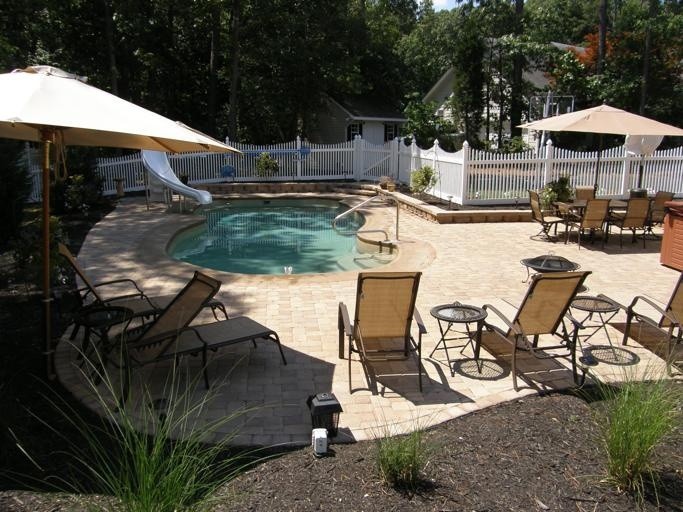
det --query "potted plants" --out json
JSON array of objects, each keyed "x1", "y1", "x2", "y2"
[{"x1": 378, "y1": 174, "x2": 395, "y2": 192}]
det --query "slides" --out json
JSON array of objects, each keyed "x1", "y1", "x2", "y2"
[{"x1": 142, "y1": 150, "x2": 212, "y2": 206}]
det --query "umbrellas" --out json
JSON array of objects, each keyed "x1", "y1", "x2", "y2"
[
  {"x1": 515, "y1": 98, "x2": 683, "y2": 198},
  {"x1": 1, "y1": 65, "x2": 242, "y2": 351}
]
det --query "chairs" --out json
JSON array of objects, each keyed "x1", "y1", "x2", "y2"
[
  {"x1": 337, "y1": 271, "x2": 428, "y2": 397},
  {"x1": 56, "y1": 240, "x2": 230, "y2": 378},
  {"x1": 593, "y1": 269, "x2": 683, "y2": 375},
  {"x1": 115, "y1": 271, "x2": 288, "y2": 406},
  {"x1": 527, "y1": 185, "x2": 675, "y2": 250},
  {"x1": 216, "y1": 165, "x2": 236, "y2": 184},
  {"x1": 477, "y1": 270, "x2": 593, "y2": 392}
]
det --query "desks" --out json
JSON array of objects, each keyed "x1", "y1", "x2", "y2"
[
  {"x1": 570, "y1": 295, "x2": 620, "y2": 362},
  {"x1": 428, "y1": 303, "x2": 488, "y2": 377}
]
